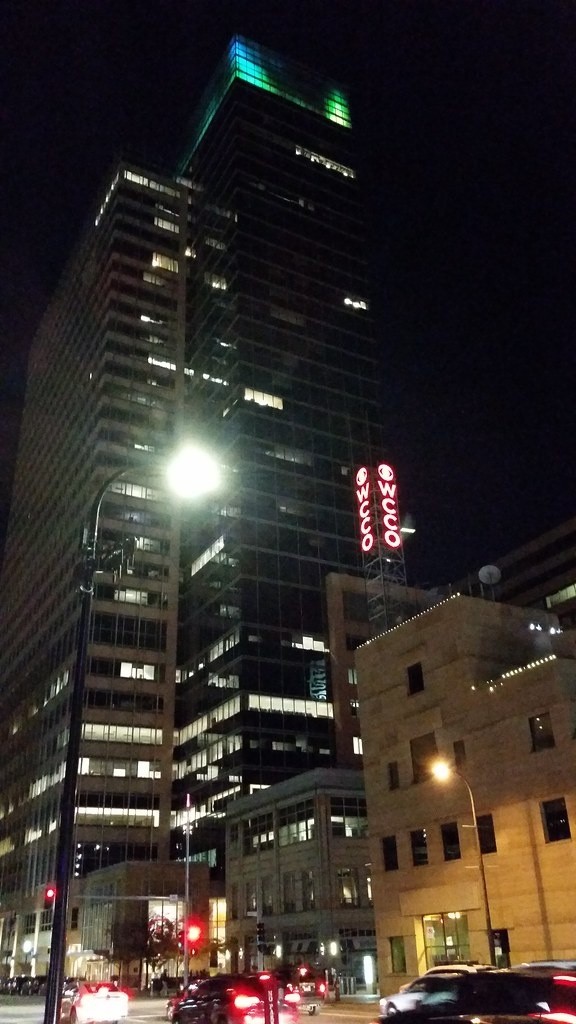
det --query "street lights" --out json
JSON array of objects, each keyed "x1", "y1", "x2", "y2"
[
  {"x1": 431, "y1": 764, "x2": 496, "y2": 968},
  {"x1": 44, "y1": 448, "x2": 220, "y2": 1024}
]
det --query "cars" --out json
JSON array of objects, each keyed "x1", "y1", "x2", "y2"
[
  {"x1": 61, "y1": 981, "x2": 128, "y2": 1024},
  {"x1": 166, "y1": 963, "x2": 327, "y2": 1024},
  {"x1": 370, "y1": 960, "x2": 575, "y2": 1024},
  {"x1": 1, "y1": 975, "x2": 87, "y2": 996}
]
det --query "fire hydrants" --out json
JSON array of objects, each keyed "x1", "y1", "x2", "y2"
[{"x1": 334, "y1": 980, "x2": 340, "y2": 1001}]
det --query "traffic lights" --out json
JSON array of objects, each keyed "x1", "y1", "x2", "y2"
[
  {"x1": 189, "y1": 947, "x2": 196, "y2": 957},
  {"x1": 256, "y1": 922, "x2": 264, "y2": 940},
  {"x1": 187, "y1": 921, "x2": 200, "y2": 940},
  {"x1": 44, "y1": 885, "x2": 53, "y2": 909},
  {"x1": 256, "y1": 943, "x2": 277, "y2": 956}
]
